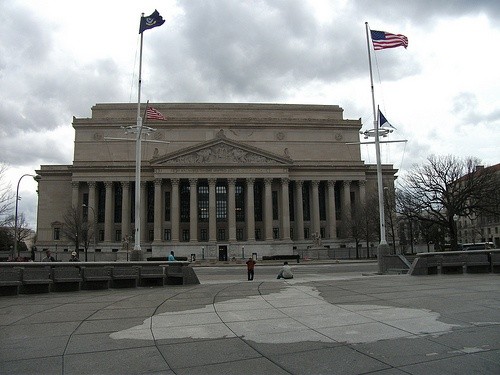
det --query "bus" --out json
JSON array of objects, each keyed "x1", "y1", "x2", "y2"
[{"x1": 439, "y1": 243, "x2": 496, "y2": 252}]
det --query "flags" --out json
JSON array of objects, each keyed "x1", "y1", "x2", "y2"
[
  {"x1": 139, "y1": 9, "x2": 165, "y2": 34},
  {"x1": 146, "y1": 106, "x2": 167, "y2": 120},
  {"x1": 371, "y1": 30, "x2": 408, "y2": 50}
]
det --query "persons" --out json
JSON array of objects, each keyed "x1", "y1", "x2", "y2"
[
  {"x1": 246, "y1": 257, "x2": 256, "y2": 281},
  {"x1": 277, "y1": 261, "x2": 293, "y2": 279},
  {"x1": 42, "y1": 250, "x2": 56, "y2": 262},
  {"x1": 168, "y1": 251, "x2": 176, "y2": 261},
  {"x1": 314, "y1": 230, "x2": 321, "y2": 246},
  {"x1": 124, "y1": 233, "x2": 130, "y2": 250},
  {"x1": 68, "y1": 252, "x2": 80, "y2": 262}
]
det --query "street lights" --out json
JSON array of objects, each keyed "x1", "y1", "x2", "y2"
[
  {"x1": 12, "y1": 173, "x2": 43, "y2": 257},
  {"x1": 81, "y1": 204, "x2": 96, "y2": 261}
]
